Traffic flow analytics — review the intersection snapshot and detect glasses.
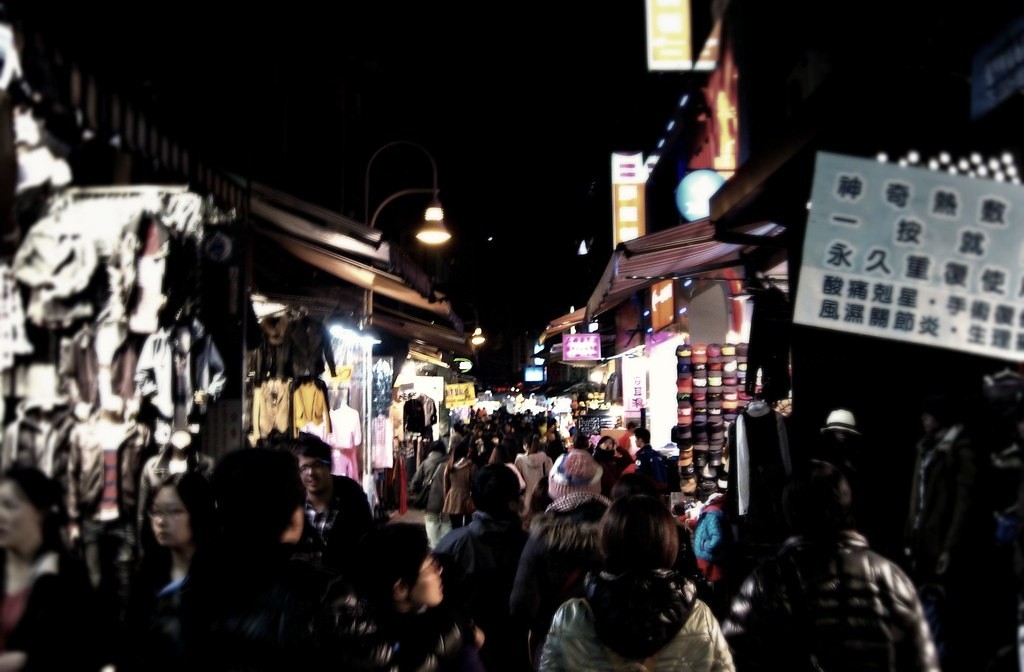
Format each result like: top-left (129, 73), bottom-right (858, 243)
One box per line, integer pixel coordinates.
top-left (298, 460), bottom-right (332, 472)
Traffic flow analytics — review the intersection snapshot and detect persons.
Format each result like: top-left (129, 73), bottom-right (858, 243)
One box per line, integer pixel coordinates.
top-left (138, 430), bottom-right (215, 560)
top-left (0, 404), bottom-right (1023, 671)
top-left (65, 395), bottom-right (152, 609)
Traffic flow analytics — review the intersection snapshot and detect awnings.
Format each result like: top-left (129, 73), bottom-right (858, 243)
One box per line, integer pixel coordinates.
top-left (584, 215), bottom-right (788, 324)
top-left (538, 305), bottom-right (626, 346)
top-left (242, 182), bottom-right (475, 355)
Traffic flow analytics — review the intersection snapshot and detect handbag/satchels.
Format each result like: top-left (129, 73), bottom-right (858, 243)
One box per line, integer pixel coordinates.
top-left (414, 483), bottom-right (431, 509)
top-left (464, 497), bottom-right (475, 512)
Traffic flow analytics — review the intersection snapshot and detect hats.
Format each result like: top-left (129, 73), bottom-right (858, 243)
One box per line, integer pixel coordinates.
top-left (548, 451), bottom-right (605, 499)
top-left (820, 409), bottom-right (864, 439)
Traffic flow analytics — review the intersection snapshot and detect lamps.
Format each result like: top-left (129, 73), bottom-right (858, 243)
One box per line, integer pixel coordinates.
top-left (366, 140), bottom-right (453, 248)
